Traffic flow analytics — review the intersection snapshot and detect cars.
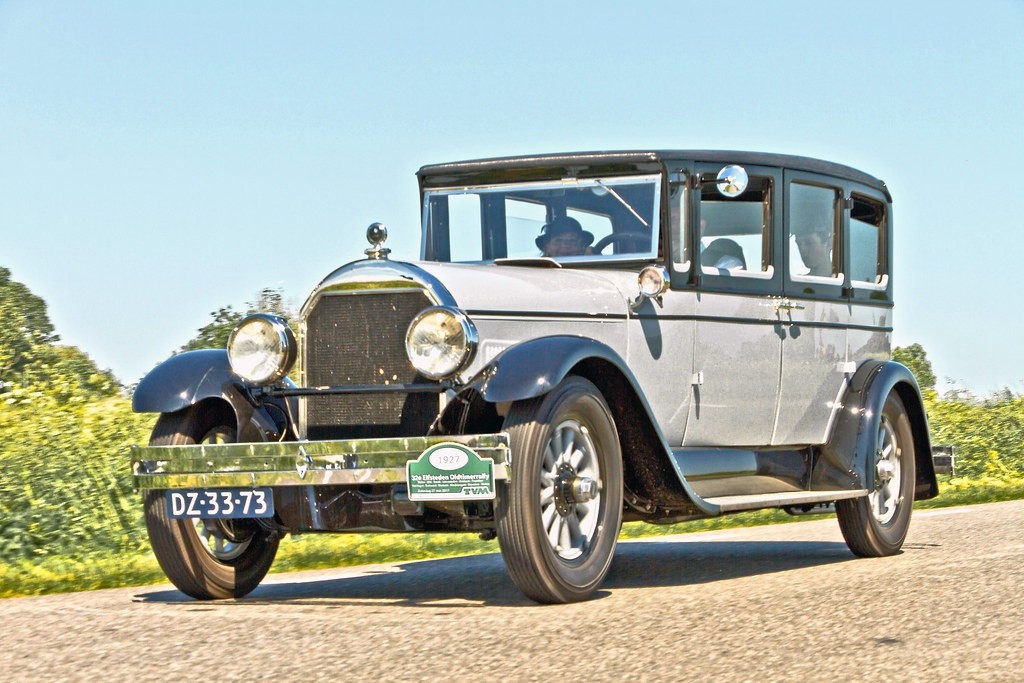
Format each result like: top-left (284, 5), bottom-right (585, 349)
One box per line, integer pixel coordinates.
top-left (128, 148), bottom-right (940, 606)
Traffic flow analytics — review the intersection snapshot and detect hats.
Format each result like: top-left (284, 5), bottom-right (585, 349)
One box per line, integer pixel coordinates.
top-left (535, 217), bottom-right (593, 253)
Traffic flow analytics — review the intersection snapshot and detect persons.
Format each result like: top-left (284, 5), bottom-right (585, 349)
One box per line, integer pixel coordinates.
top-left (790, 206), bottom-right (834, 277)
top-left (670, 195), bottom-right (744, 270)
top-left (535, 216), bottom-right (602, 257)
